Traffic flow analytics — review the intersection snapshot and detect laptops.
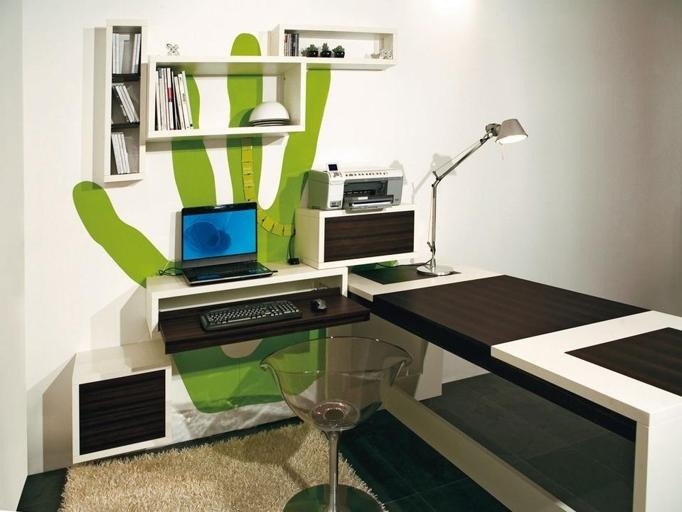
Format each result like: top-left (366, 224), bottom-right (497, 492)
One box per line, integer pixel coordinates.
top-left (181, 202), bottom-right (273, 286)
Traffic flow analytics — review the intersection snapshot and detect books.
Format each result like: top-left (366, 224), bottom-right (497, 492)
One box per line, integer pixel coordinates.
top-left (155, 66), bottom-right (194, 133)
top-left (111, 31), bottom-right (142, 176)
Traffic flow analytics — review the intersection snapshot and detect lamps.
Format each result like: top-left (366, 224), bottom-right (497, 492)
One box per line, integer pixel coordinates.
top-left (416, 118), bottom-right (528, 276)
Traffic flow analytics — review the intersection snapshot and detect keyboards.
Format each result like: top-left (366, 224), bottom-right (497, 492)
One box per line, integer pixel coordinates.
top-left (200, 300), bottom-right (303, 331)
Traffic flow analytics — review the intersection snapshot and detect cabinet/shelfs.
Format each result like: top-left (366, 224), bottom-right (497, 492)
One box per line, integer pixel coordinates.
top-left (146, 54), bottom-right (307, 143)
top-left (70, 330), bottom-right (320, 465)
top-left (270, 22), bottom-right (399, 72)
top-left (145, 263), bottom-right (371, 355)
top-left (294, 206), bottom-right (681, 512)
top-left (100, 16), bottom-right (149, 184)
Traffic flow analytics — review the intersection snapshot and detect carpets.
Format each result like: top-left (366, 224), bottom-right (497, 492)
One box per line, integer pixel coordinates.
top-left (57, 417), bottom-right (389, 512)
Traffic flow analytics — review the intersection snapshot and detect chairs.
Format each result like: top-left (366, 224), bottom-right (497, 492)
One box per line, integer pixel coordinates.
top-left (260, 335), bottom-right (413, 512)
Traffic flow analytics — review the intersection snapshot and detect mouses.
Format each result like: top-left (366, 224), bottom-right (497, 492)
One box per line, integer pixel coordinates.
top-left (313, 298), bottom-right (328, 313)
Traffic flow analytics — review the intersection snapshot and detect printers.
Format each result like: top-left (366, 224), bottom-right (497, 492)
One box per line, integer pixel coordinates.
top-left (307, 164), bottom-right (404, 213)
